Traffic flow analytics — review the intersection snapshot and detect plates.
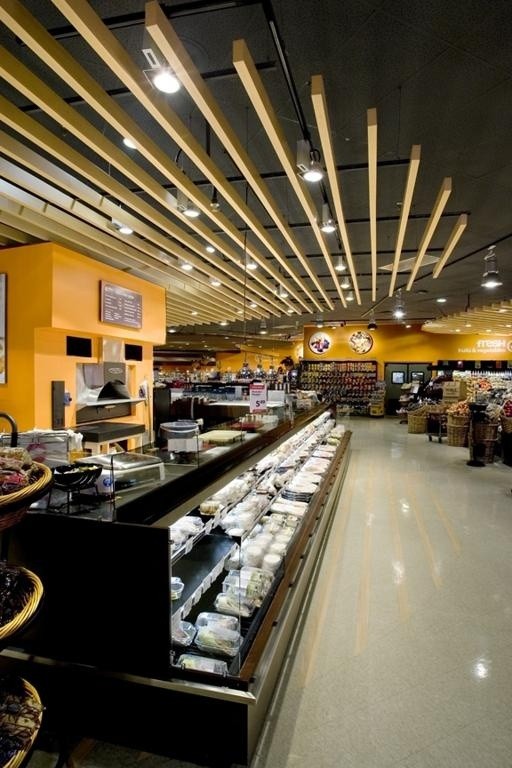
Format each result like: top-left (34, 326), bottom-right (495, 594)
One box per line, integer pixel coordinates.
top-left (270, 444), bottom-right (342, 515)
top-left (228, 422), bottom-right (267, 433)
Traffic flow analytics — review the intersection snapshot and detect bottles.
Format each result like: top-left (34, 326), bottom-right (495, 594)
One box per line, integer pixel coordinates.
top-left (96, 474), bottom-right (115, 493)
top-left (307, 361), bottom-right (373, 372)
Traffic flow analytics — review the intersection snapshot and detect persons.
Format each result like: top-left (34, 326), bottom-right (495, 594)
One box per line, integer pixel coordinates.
top-left (277, 356), bottom-right (299, 390)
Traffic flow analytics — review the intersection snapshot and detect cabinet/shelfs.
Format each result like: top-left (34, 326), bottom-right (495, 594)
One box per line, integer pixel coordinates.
top-left (300, 357), bottom-right (378, 416)
top-left (162, 411), bottom-right (353, 685)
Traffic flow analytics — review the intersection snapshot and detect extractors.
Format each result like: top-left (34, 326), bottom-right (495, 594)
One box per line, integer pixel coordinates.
top-left (79, 364), bottom-right (146, 407)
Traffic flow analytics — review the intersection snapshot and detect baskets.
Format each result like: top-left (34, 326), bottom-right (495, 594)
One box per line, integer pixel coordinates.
top-left (446, 412), bottom-right (512, 463)
top-left (1, 460), bottom-right (48, 529)
top-left (0, 567), bottom-right (43, 652)
top-left (401, 388), bottom-right (411, 395)
top-left (398, 413), bottom-right (408, 420)
top-left (407, 414), bottom-right (428, 433)
top-left (0, 675), bottom-right (43, 768)
top-left (52, 463), bottom-right (103, 488)
top-left (398, 400), bottom-right (409, 407)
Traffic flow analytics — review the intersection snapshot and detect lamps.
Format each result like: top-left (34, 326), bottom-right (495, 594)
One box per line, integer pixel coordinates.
top-left (480, 245), bottom-right (504, 291)
top-left (112, 191), bottom-right (289, 301)
top-left (302, 140), bottom-right (326, 183)
top-left (389, 289), bottom-right (408, 319)
top-left (366, 311), bottom-right (378, 332)
top-left (319, 204), bottom-right (338, 234)
top-left (334, 253), bottom-right (356, 303)
top-left (235, 352), bottom-right (276, 382)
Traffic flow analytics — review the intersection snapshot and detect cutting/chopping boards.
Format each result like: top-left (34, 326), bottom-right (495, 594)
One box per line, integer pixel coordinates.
top-left (196, 429), bottom-right (248, 444)
